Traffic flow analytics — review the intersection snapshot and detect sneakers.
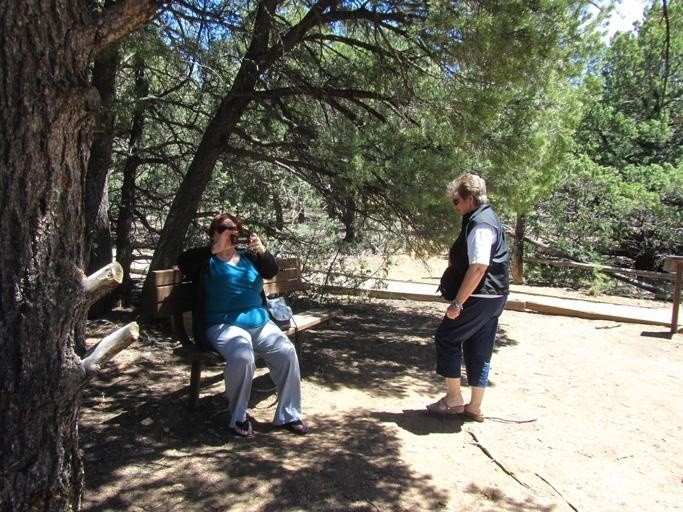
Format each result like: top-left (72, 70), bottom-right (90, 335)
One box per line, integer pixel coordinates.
top-left (282, 418), bottom-right (309, 433)
top-left (427, 398), bottom-right (480, 418)
top-left (235, 416), bottom-right (253, 436)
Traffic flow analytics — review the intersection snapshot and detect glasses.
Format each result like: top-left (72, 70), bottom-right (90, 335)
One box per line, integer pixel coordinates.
top-left (452, 197), bottom-right (462, 205)
top-left (214, 225), bottom-right (237, 234)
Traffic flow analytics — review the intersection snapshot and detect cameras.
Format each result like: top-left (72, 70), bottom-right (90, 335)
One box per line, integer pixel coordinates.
top-left (231, 234), bottom-right (251, 244)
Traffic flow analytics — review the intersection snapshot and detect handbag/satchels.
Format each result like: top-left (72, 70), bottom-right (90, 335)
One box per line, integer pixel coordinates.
top-left (266, 296), bottom-right (293, 331)
top-left (439, 264), bottom-right (487, 301)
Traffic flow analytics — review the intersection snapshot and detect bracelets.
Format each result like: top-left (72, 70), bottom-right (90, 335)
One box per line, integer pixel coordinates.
top-left (453, 299), bottom-right (464, 311)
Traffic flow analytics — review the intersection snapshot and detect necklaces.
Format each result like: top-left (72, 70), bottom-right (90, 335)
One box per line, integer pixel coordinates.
top-left (219, 250), bottom-right (235, 262)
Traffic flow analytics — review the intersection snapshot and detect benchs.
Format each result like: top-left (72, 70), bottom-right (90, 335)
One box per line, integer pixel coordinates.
top-left (142, 257), bottom-right (343, 413)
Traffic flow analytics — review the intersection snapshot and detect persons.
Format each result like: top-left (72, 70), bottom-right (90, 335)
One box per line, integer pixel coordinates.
top-left (425, 173), bottom-right (510, 422)
top-left (175, 212), bottom-right (310, 438)
